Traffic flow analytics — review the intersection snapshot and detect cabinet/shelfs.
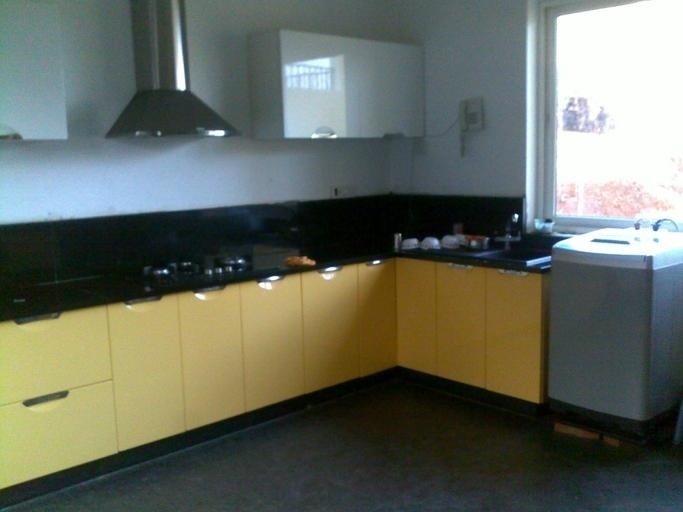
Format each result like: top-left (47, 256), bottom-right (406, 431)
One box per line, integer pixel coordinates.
top-left (250, 27), bottom-right (362, 141)
top-left (359, 256), bottom-right (396, 381)
top-left (245, 261), bottom-right (362, 416)
top-left (1, 306), bottom-right (118, 492)
top-left (391, 255), bottom-right (436, 377)
top-left (362, 36), bottom-right (426, 142)
top-left (0, 0), bottom-right (69, 140)
top-left (437, 259), bottom-right (549, 404)
top-left (108, 279), bottom-right (243, 455)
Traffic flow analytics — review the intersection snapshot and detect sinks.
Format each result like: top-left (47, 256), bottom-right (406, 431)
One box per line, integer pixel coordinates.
top-left (475, 245), bottom-right (554, 264)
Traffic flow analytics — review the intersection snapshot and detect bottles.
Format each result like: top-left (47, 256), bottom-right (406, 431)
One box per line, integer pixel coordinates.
top-left (393, 232), bottom-right (402, 250)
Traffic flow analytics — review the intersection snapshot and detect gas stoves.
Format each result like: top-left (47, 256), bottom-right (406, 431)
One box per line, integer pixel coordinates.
top-left (123, 247), bottom-right (277, 298)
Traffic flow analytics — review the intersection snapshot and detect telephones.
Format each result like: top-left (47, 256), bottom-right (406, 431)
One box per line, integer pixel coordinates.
top-left (458, 96), bottom-right (483, 132)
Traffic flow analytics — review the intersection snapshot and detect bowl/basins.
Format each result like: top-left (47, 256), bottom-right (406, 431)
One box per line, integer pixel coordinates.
top-left (534, 217), bottom-right (556, 234)
top-left (466, 235), bottom-right (489, 250)
top-left (400, 236), bottom-right (419, 251)
top-left (441, 234), bottom-right (459, 250)
top-left (420, 236), bottom-right (442, 250)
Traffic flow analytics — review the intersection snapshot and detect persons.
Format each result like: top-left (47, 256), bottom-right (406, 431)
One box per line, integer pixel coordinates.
top-left (566, 96), bottom-right (609, 134)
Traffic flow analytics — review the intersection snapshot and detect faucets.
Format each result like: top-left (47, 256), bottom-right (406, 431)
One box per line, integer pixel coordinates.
top-left (503, 234), bottom-right (512, 255)
